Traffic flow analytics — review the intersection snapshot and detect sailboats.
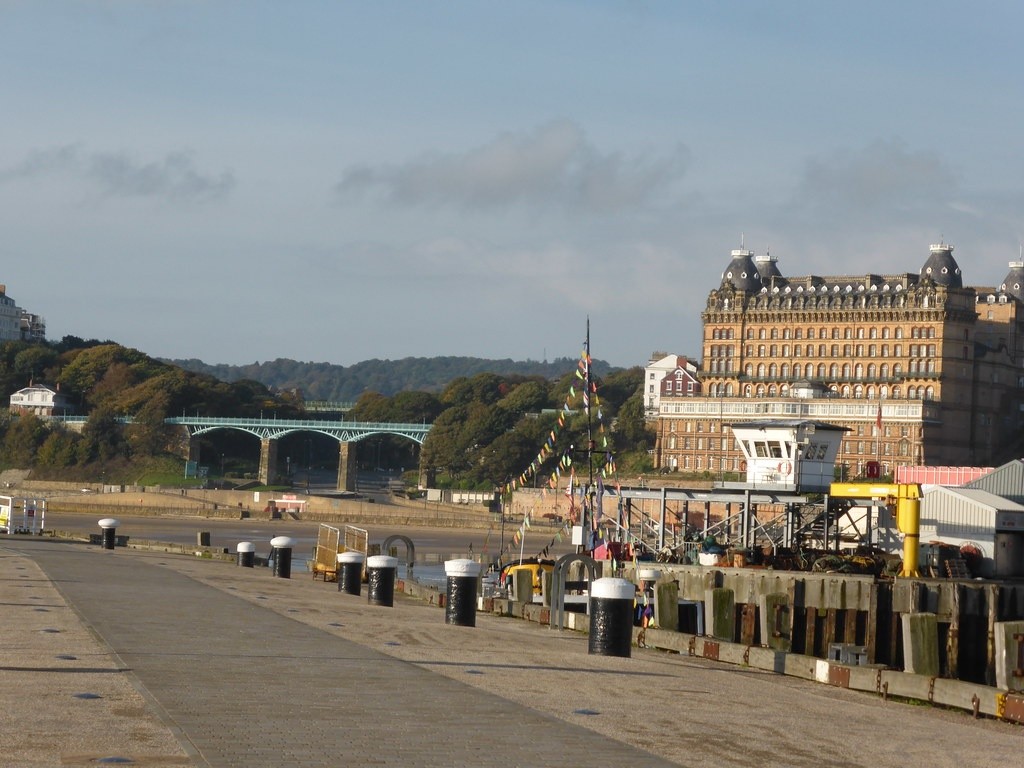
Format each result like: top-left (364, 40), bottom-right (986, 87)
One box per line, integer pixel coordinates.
top-left (498, 314), bottom-right (705, 638)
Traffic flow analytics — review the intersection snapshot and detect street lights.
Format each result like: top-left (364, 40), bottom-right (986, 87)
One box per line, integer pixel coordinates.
top-left (219, 449), bottom-right (225, 479)
top-left (260, 409), bottom-right (263, 419)
top-left (341, 414), bottom-right (344, 421)
top-left (304, 435), bottom-right (313, 494)
top-left (274, 411), bottom-right (276, 419)
top-left (377, 438), bottom-right (383, 472)
top-left (182, 408), bottom-right (186, 422)
top-left (354, 413), bottom-right (357, 422)
top-left (197, 409), bottom-right (199, 418)
top-left (423, 415), bottom-right (426, 424)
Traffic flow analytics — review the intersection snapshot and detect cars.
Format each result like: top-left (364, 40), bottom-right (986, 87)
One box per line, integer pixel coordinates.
top-left (543, 514), bottom-right (563, 523)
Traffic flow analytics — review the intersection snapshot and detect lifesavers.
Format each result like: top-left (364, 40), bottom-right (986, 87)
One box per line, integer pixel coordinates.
top-left (777, 460), bottom-right (791, 477)
top-left (740, 461), bottom-right (747, 471)
